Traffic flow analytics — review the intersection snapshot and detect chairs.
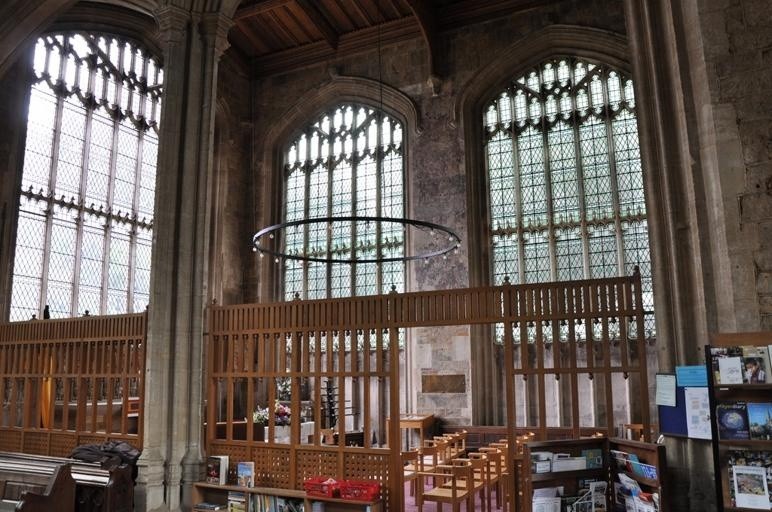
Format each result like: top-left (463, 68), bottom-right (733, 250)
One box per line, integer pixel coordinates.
top-left (402, 429), bottom-right (536, 512)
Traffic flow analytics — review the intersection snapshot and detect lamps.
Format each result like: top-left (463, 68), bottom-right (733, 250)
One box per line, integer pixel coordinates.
top-left (250, 0), bottom-right (461, 267)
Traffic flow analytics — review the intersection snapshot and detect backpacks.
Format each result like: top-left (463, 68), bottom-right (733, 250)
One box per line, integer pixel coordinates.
top-left (67, 438), bottom-right (139, 466)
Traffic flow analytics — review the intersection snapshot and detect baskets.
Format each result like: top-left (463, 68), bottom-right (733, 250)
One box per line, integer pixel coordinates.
top-left (303, 477), bottom-right (344, 498)
top-left (339, 481), bottom-right (380, 501)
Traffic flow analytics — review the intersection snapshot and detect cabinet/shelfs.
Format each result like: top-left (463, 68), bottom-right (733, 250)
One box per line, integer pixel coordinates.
top-left (522, 435), bottom-right (608, 512)
top-left (608, 437), bottom-right (667, 512)
top-left (191, 481), bottom-right (383, 512)
top-left (704, 330), bottom-right (772, 512)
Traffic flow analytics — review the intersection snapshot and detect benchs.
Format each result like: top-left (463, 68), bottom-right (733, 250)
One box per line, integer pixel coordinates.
top-left (0, 450), bottom-right (134, 512)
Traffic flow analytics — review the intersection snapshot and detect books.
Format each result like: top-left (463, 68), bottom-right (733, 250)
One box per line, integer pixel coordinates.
top-left (237, 461), bottom-right (255, 488)
top-left (192, 490), bottom-right (325, 512)
top-left (528, 449), bottom-right (660, 511)
top-left (207, 455), bottom-right (230, 486)
top-left (707, 345), bottom-right (772, 511)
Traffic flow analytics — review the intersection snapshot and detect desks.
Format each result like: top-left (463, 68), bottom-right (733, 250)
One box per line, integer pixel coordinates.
top-left (386, 413), bottom-right (434, 447)
